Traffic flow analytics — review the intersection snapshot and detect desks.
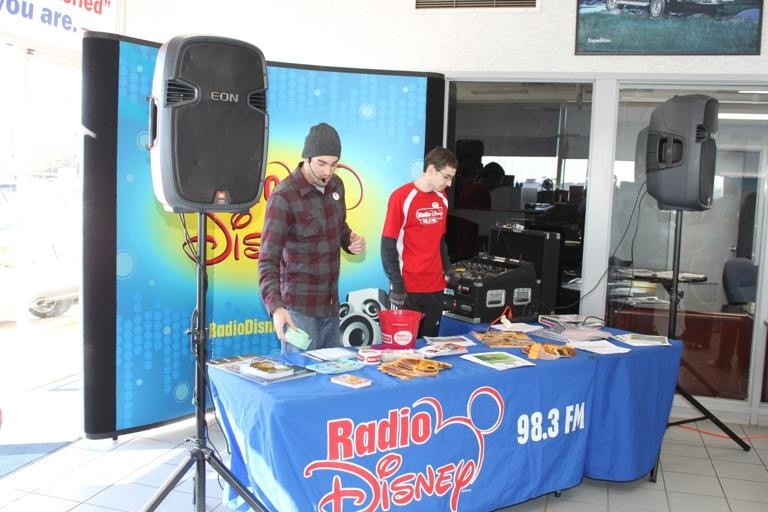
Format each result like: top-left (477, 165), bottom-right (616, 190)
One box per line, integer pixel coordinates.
top-left (207, 316), bottom-right (684, 512)
top-left (611, 304), bottom-right (768, 402)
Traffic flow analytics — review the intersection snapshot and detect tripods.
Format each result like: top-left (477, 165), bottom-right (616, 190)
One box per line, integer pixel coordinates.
top-left (635, 214), bottom-right (753, 483)
top-left (141, 218), bottom-right (271, 512)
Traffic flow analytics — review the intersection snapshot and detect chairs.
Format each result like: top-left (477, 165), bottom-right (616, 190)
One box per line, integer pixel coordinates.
top-left (723, 254), bottom-right (759, 306)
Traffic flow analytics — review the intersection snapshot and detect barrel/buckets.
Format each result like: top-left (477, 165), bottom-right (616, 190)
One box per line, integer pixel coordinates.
top-left (378, 310), bottom-right (426, 349)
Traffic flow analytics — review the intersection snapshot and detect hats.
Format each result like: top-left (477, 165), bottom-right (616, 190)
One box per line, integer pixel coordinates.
top-left (302, 123), bottom-right (341, 158)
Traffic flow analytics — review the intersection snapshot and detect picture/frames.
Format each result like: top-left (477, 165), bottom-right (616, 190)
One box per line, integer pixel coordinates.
top-left (574, 0), bottom-right (762, 54)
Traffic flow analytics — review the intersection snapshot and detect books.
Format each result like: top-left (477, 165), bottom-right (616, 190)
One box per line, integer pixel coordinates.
top-left (239, 360), bottom-right (295, 380)
top-left (330, 373), bottom-right (371, 390)
top-left (628, 333), bottom-right (665, 344)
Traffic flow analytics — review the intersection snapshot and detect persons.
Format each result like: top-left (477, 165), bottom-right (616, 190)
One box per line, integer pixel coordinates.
top-left (450, 138), bottom-right (503, 262)
top-left (381, 146), bottom-right (460, 341)
top-left (257, 123), bottom-right (366, 356)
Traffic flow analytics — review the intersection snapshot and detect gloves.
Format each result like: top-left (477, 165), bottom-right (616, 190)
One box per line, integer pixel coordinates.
top-left (443, 267), bottom-right (459, 287)
top-left (388, 280), bottom-right (407, 309)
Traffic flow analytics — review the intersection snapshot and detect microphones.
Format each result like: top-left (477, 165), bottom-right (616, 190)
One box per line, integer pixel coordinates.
top-left (306, 158), bottom-right (327, 188)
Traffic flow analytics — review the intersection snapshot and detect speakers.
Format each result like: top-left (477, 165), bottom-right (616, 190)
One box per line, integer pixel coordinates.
top-left (337, 288), bottom-right (391, 348)
top-left (643, 95), bottom-right (721, 211)
top-left (147, 32), bottom-right (271, 214)
